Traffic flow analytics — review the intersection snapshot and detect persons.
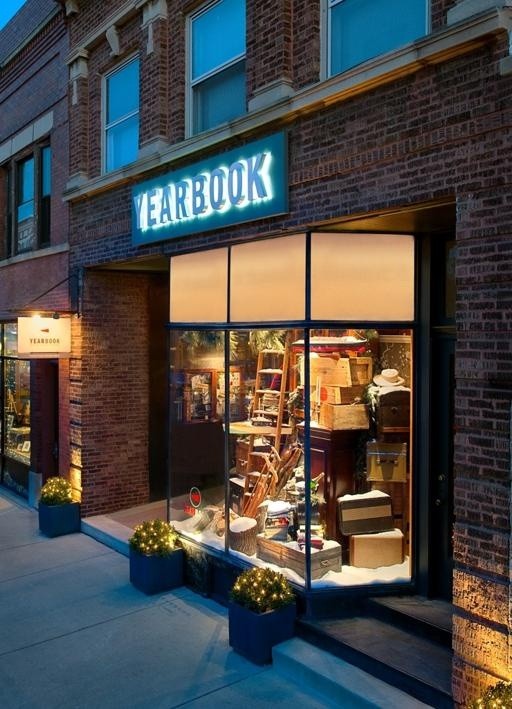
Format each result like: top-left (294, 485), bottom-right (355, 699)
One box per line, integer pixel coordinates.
top-left (18, 398), bottom-right (30, 429)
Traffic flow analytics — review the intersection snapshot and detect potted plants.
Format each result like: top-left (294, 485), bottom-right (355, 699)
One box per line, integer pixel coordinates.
top-left (226, 565), bottom-right (298, 666)
top-left (127, 515), bottom-right (186, 597)
top-left (36, 474), bottom-right (82, 538)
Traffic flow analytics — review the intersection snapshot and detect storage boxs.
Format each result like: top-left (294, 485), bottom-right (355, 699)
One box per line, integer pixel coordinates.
top-left (224, 341), bottom-right (411, 580)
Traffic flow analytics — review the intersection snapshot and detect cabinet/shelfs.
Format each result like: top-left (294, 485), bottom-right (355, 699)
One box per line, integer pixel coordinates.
top-left (1, 356), bottom-right (61, 500)
top-left (178, 364), bottom-right (246, 427)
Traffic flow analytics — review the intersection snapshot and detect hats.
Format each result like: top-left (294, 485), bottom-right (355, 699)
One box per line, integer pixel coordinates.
top-left (373, 369), bottom-right (405, 386)
